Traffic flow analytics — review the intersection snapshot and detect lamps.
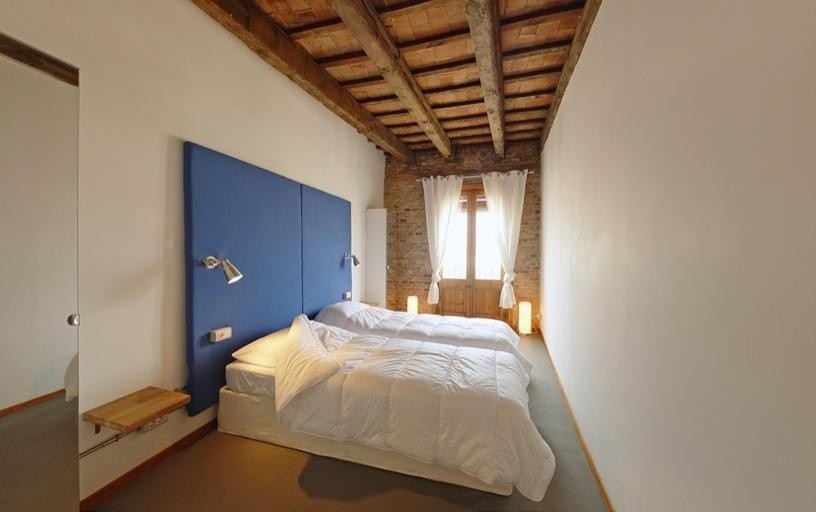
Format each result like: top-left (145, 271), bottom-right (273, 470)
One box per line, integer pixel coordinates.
top-left (407, 296), bottom-right (420, 314)
top-left (518, 302), bottom-right (532, 334)
top-left (344, 254), bottom-right (362, 267)
top-left (204, 255), bottom-right (243, 286)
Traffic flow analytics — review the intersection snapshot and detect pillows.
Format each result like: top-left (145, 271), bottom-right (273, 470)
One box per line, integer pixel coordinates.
top-left (231, 325), bottom-right (291, 368)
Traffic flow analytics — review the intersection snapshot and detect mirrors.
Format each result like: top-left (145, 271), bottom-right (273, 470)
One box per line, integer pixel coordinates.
top-left (0, 32), bottom-right (82, 512)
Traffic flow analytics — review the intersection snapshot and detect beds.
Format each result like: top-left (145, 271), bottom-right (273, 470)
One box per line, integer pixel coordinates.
top-left (313, 301), bottom-right (534, 381)
top-left (214, 315), bottom-right (556, 502)
